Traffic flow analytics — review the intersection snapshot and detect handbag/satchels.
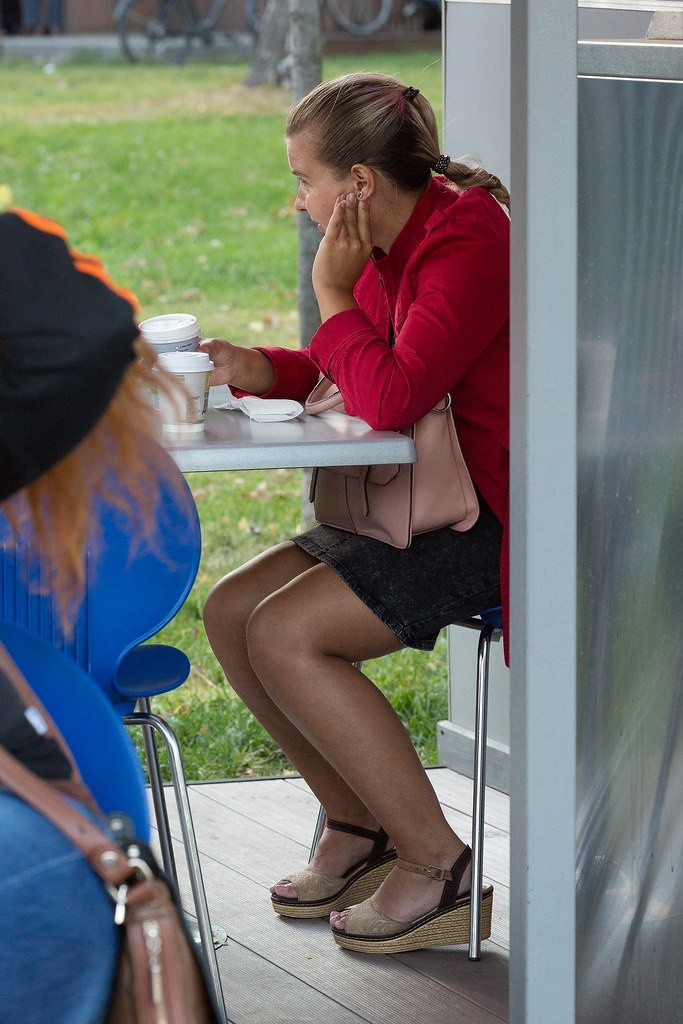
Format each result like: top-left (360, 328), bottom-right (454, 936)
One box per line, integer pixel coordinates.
top-left (90, 839), bottom-right (212, 1024)
top-left (304, 375), bottom-right (479, 550)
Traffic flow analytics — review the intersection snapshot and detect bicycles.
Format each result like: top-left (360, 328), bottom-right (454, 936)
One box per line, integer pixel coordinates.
top-left (117, 1), bottom-right (267, 67)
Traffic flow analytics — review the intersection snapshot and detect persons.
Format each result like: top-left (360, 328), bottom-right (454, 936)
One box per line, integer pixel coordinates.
top-left (0, 208), bottom-right (190, 1024)
top-left (196, 75), bottom-right (510, 954)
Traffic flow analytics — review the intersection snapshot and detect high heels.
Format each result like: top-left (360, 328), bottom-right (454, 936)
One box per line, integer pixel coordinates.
top-left (270, 816), bottom-right (398, 918)
top-left (328, 843), bottom-right (494, 954)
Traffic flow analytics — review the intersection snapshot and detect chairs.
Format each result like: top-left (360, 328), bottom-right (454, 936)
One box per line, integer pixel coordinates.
top-left (0, 425), bottom-right (228, 1024)
top-left (303, 604), bottom-right (507, 958)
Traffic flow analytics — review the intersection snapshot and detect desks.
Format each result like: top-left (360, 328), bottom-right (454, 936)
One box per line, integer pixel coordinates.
top-left (134, 417), bottom-right (420, 962)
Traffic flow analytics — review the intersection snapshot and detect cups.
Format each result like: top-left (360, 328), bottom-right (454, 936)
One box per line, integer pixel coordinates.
top-left (138, 312), bottom-right (202, 412)
top-left (151, 351), bottom-right (215, 433)
